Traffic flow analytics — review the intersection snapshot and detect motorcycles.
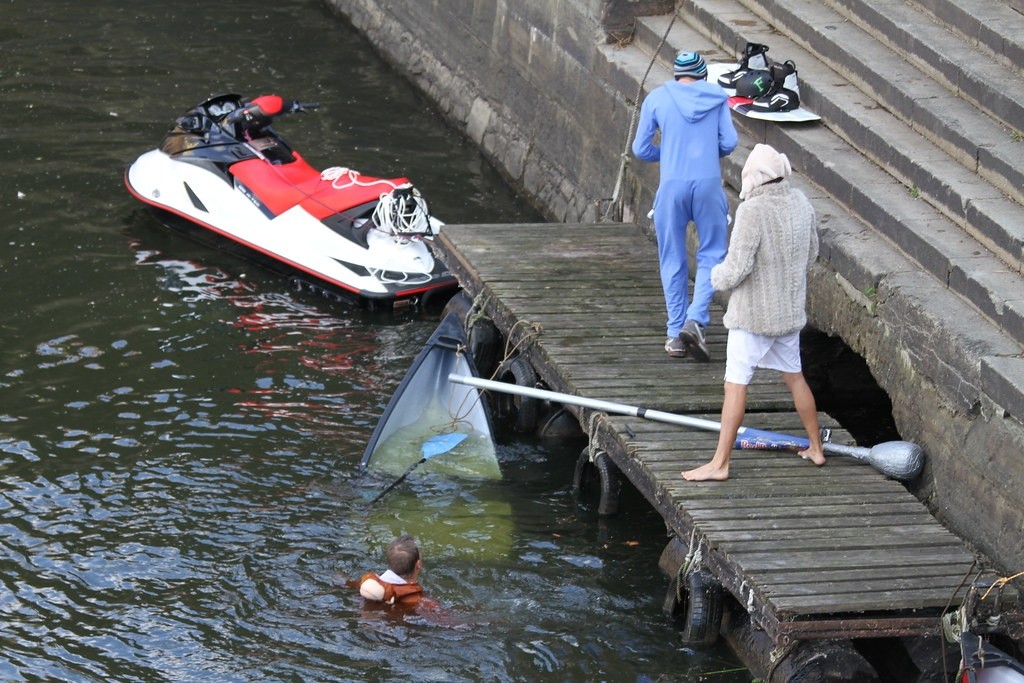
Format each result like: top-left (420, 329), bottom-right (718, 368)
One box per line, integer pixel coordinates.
top-left (123, 91), bottom-right (462, 314)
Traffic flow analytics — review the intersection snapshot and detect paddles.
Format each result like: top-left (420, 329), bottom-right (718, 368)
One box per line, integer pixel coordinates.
top-left (366, 432), bottom-right (470, 505)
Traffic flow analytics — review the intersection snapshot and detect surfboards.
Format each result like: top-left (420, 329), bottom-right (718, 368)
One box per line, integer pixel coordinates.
top-left (704, 64), bottom-right (823, 123)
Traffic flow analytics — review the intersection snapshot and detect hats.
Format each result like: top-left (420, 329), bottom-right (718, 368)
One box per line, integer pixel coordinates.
top-left (739, 143), bottom-right (792, 199)
top-left (674, 51), bottom-right (708, 81)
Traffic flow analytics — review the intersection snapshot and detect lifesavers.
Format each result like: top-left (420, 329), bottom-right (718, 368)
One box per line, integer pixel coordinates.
top-left (572, 446), bottom-right (624, 523)
top-left (460, 311), bottom-right (501, 376)
top-left (661, 563), bottom-right (725, 649)
top-left (490, 359), bottom-right (543, 429)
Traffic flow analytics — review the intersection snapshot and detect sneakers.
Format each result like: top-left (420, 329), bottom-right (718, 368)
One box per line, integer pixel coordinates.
top-left (679, 320), bottom-right (711, 361)
top-left (718, 42), bottom-right (769, 88)
top-left (664, 337), bottom-right (688, 357)
top-left (752, 60), bottom-right (800, 112)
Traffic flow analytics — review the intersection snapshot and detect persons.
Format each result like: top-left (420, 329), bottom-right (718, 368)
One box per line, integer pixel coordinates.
top-left (632, 51), bottom-right (738, 361)
top-left (682, 143), bottom-right (826, 480)
top-left (359, 535), bottom-right (424, 603)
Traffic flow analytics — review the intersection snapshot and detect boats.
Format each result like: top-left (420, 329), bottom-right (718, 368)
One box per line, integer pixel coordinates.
top-left (348, 306), bottom-right (518, 566)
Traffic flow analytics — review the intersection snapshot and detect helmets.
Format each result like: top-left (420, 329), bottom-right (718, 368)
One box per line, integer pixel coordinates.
top-left (736, 70), bottom-right (776, 99)
